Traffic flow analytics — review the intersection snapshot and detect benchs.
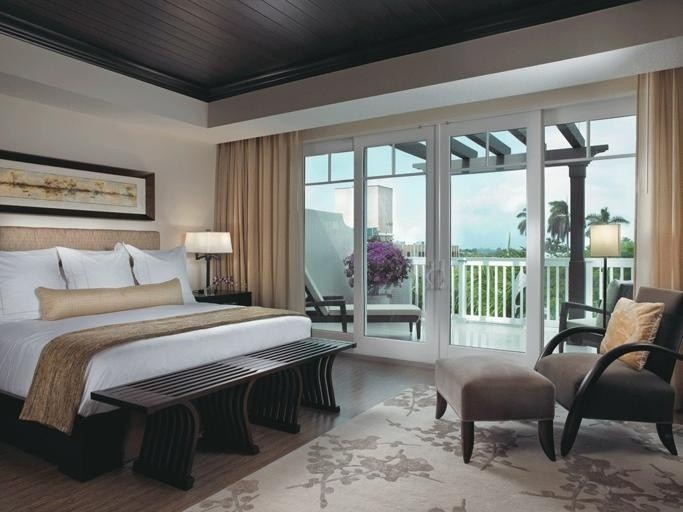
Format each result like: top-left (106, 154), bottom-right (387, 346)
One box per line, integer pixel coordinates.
top-left (91, 337), bottom-right (356, 490)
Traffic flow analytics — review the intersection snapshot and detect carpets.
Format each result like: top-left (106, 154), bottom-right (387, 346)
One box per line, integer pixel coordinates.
top-left (182, 383), bottom-right (683, 512)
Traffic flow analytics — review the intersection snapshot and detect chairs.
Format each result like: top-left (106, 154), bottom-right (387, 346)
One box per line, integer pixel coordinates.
top-left (535, 287), bottom-right (683, 456)
top-left (303, 269), bottom-right (422, 340)
top-left (559, 282), bottom-right (633, 353)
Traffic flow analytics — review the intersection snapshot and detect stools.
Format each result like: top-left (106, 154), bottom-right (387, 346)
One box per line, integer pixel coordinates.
top-left (434, 354), bottom-right (554, 463)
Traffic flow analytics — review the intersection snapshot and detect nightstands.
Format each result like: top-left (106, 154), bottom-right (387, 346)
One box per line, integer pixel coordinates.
top-left (193, 290), bottom-right (251, 306)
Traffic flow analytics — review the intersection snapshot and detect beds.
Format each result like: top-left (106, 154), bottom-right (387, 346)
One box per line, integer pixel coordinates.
top-left (0, 227), bottom-right (312, 482)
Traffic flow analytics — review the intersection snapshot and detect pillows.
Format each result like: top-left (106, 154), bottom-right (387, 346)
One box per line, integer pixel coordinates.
top-left (36, 278), bottom-right (183, 322)
top-left (123, 241), bottom-right (197, 305)
top-left (600, 297), bottom-right (665, 371)
top-left (596, 280), bottom-right (632, 329)
top-left (0, 248), bottom-right (65, 321)
top-left (58, 242), bottom-right (136, 290)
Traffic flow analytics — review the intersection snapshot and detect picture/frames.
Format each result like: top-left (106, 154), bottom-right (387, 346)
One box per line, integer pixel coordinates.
top-left (0, 149), bottom-right (154, 220)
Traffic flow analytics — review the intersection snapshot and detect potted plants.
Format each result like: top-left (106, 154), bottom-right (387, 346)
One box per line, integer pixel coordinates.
top-left (344, 241), bottom-right (409, 304)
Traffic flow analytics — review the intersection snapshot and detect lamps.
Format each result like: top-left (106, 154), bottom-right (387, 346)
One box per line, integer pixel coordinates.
top-left (184, 228), bottom-right (233, 294)
top-left (590, 223), bottom-right (618, 330)
